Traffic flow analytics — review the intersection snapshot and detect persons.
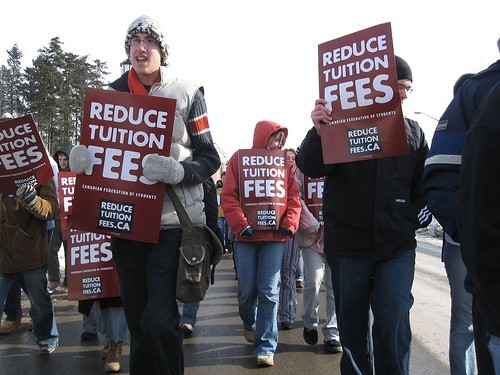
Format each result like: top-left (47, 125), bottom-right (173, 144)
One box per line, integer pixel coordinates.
top-left (46, 145), bottom-right (73, 293)
top-left (221, 121), bottom-right (301, 366)
top-left (292, 164), bottom-right (342, 353)
top-left (0, 113), bottom-right (59, 354)
top-left (178, 176), bottom-right (219, 335)
top-left (70, 14), bottom-right (221, 375)
top-left (426, 38), bottom-right (500, 375)
top-left (216, 171), bottom-right (232, 257)
top-left (295, 54), bottom-right (430, 375)
top-left (78, 296), bottom-right (132, 373)
top-left (276, 147), bottom-right (306, 330)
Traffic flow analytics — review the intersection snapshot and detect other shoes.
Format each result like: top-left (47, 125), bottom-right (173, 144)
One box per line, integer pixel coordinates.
top-left (283, 325), bottom-right (293, 330)
top-left (64, 278), bottom-right (67, 287)
top-left (303, 327), bottom-right (318, 345)
top-left (0, 320), bottom-right (21, 334)
top-left (81, 332), bottom-right (97, 341)
top-left (295, 281), bottom-right (303, 289)
top-left (102, 343), bottom-right (112, 359)
top-left (324, 340), bottom-right (343, 352)
top-left (39, 346), bottom-right (55, 354)
top-left (104, 342), bottom-right (122, 372)
top-left (182, 323), bottom-right (193, 336)
top-left (47, 281), bottom-right (60, 294)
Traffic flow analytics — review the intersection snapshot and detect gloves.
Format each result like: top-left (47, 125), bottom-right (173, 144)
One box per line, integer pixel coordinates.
top-left (69, 145), bottom-right (90, 174)
top-left (239, 226), bottom-right (254, 237)
top-left (143, 154), bottom-right (185, 185)
top-left (278, 228), bottom-right (292, 236)
top-left (16, 185), bottom-right (37, 204)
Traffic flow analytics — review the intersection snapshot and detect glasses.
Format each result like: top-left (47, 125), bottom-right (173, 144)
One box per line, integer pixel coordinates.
top-left (398, 85), bottom-right (413, 94)
top-left (128, 36), bottom-right (158, 47)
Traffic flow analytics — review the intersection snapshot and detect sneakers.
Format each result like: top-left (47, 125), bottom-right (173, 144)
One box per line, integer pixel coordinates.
top-left (244, 329), bottom-right (254, 342)
top-left (256, 354), bottom-right (274, 366)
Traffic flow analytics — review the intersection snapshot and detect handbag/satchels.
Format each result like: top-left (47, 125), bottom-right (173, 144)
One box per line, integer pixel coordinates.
top-left (176, 223), bottom-right (224, 305)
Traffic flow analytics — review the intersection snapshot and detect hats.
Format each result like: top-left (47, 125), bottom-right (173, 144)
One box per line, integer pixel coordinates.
top-left (453, 74), bottom-right (476, 97)
top-left (216, 180), bottom-right (223, 188)
top-left (120, 15), bottom-right (170, 67)
top-left (395, 55), bottom-right (413, 83)
